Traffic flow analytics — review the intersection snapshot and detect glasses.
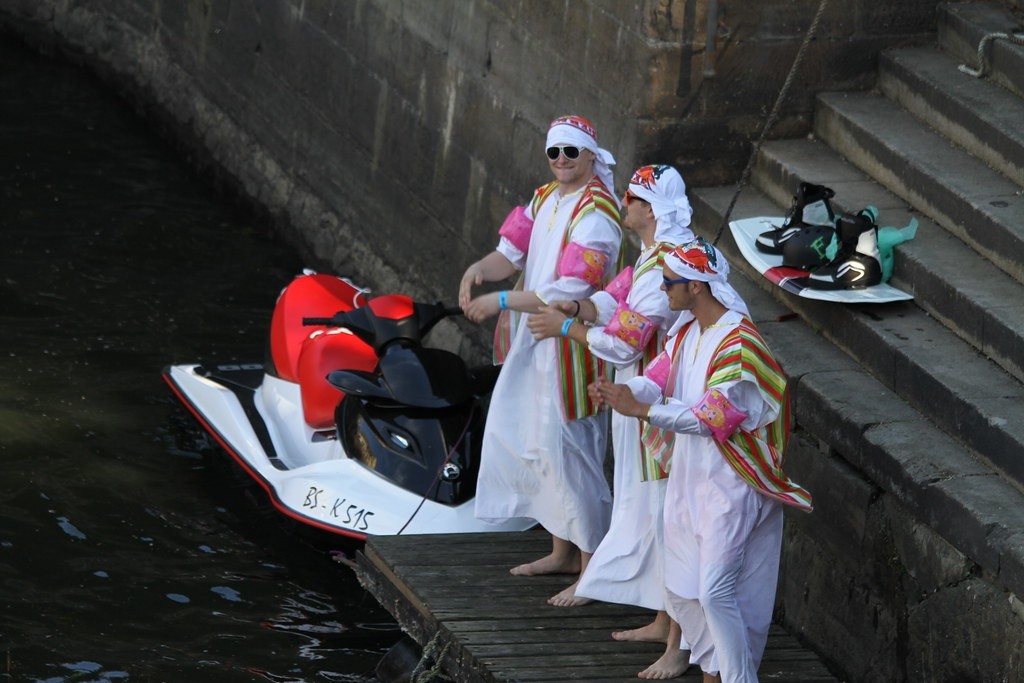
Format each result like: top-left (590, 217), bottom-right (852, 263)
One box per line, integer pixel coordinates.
top-left (625, 189), bottom-right (647, 204)
top-left (663, 274), bottom-right (693, 287)
top-left (545, 145), bottom-right (585, 161)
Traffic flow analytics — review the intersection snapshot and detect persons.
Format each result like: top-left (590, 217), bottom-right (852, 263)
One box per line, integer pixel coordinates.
top-left (588, 240), bottom-right (815, 683)
top-left (459, 115), bottom-right (624, 607)
top-left (527, 165), bottom-right (695, 680)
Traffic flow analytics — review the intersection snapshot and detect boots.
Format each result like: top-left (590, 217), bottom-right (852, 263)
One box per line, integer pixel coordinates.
top-left (806, 209), bottom-right (883, 291)
top-left (755, 179), bottom-right (836, 255)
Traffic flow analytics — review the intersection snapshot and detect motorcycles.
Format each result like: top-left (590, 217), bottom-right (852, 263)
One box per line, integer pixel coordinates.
top-left (160, 264), bottom-right (543, 552)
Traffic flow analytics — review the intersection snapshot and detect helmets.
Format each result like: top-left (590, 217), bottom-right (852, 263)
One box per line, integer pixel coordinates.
top-left (782, 226), bottom-right (841, 272)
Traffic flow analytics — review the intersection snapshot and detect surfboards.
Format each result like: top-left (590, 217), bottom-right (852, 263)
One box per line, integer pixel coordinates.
top-left (729, 215), bottom-right (915, 303)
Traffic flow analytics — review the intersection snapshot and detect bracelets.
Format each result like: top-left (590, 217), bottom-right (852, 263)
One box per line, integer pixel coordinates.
top-left (499, 290), bottom-right (508, 310)
top-left (573, 300), bottom-right (580, 317)
top-left (561, 318), bottom-right (573, 336)
